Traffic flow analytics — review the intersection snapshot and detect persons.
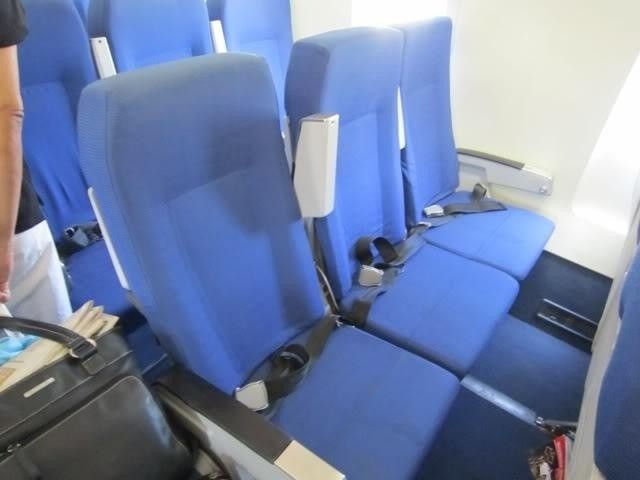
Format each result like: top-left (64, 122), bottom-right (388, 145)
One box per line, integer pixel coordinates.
top-left (1, 0), bottom-right (74, 340)
